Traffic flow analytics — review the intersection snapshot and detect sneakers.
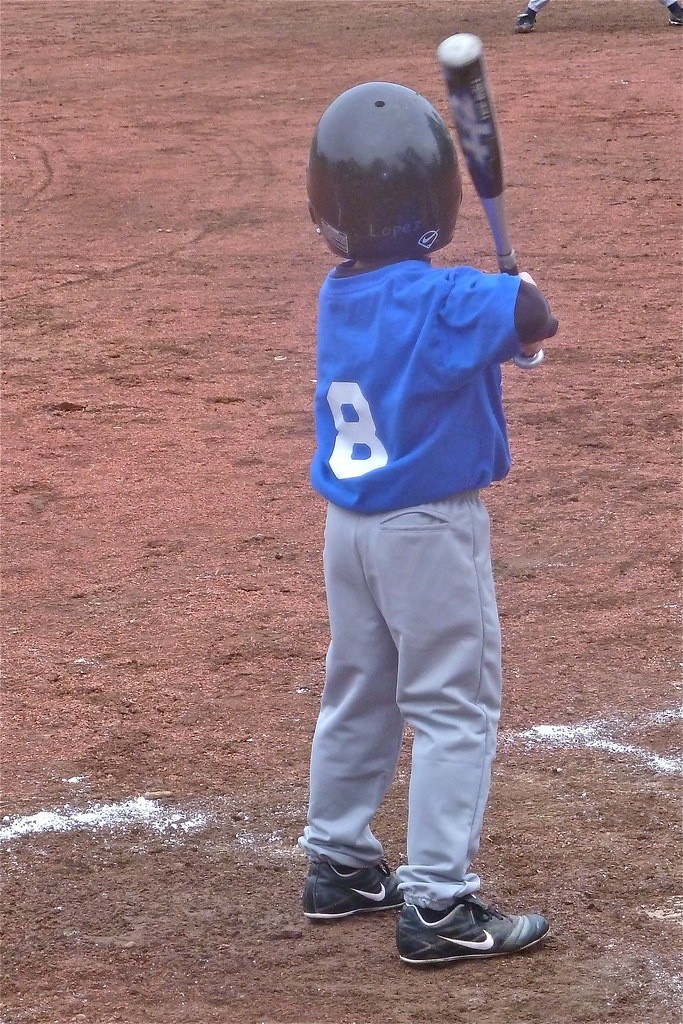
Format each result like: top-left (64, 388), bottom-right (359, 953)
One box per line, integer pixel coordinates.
top-left (301, 857), bottom-right (405, 918)
top-left (668, 14), bottom-right (683, 26)
top-left (513, 13), bottom-right (537, 33)
top-left (395, 900), bottom-right (552, 967)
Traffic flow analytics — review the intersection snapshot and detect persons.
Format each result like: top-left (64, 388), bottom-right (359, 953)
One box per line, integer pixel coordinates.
top-left (513, 0), bottom-right (682, 48)
top-left (295, 75), bottom-right (562, 966)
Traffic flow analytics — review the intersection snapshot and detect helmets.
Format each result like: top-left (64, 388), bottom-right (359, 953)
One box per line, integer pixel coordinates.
top-left (307, 82), bottom-right (464, 257)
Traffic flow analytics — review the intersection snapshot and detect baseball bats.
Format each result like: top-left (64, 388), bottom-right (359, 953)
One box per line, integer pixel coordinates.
top-left (436, 32), bottom-right (547, 371)
